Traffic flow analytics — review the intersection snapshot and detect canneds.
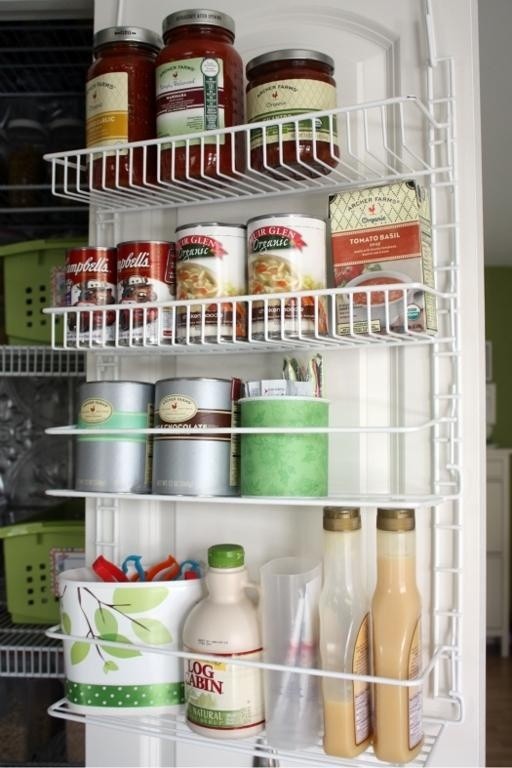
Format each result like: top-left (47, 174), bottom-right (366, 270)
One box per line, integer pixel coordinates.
top-left (247, 213), bottom-right (328, 340)
top-left (84, 27), bottom-right (164, 201)
top-left (64, 246), bottom-right (116, 347)
top-left (116, 240), bottom-right (175, 346)
top-left (153, 9), bottom-right (244, 190)
top-left (245, 47), bottom-right (340, 182)
top-left (174, 221), bottom-right (247, 344)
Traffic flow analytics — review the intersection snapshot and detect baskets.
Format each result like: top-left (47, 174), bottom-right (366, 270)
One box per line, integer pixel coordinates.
top-left (1, 239), bottom-right (88, 348)
top-left (0, 519), bottom-right (85, 624)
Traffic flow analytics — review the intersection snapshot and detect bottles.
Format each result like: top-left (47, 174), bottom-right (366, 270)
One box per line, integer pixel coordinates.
top-left (319, 507), bottom-right (372, 755)
top-left (182, 543), bottom-right (265, 733)
top-left (375, 511), bottom-right (426, 760)
